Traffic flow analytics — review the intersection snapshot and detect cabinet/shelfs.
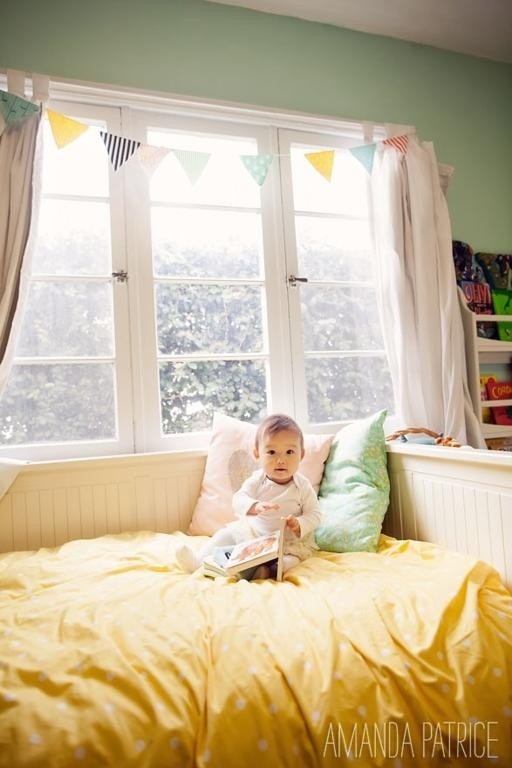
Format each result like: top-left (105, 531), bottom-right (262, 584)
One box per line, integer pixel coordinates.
top-left (457, 283), bottom-right (511, 441)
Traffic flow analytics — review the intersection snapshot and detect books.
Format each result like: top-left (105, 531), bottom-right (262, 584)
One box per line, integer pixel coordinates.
top-left (447, 236), bottom-right (512, 455)
top-left (202, 516), bottom-right (292, 583)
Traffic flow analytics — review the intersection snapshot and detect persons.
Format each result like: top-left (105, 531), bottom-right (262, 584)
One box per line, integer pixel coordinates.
top-left (200, 411), bottom-right (321, 580)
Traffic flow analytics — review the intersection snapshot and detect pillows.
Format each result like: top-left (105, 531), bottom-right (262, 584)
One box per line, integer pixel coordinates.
top-left (187, 408), bottom-right (390, 554)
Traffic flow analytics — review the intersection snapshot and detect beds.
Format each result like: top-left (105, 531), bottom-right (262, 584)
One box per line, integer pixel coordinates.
top-left (2, 442), bottom-right (512, 768)
